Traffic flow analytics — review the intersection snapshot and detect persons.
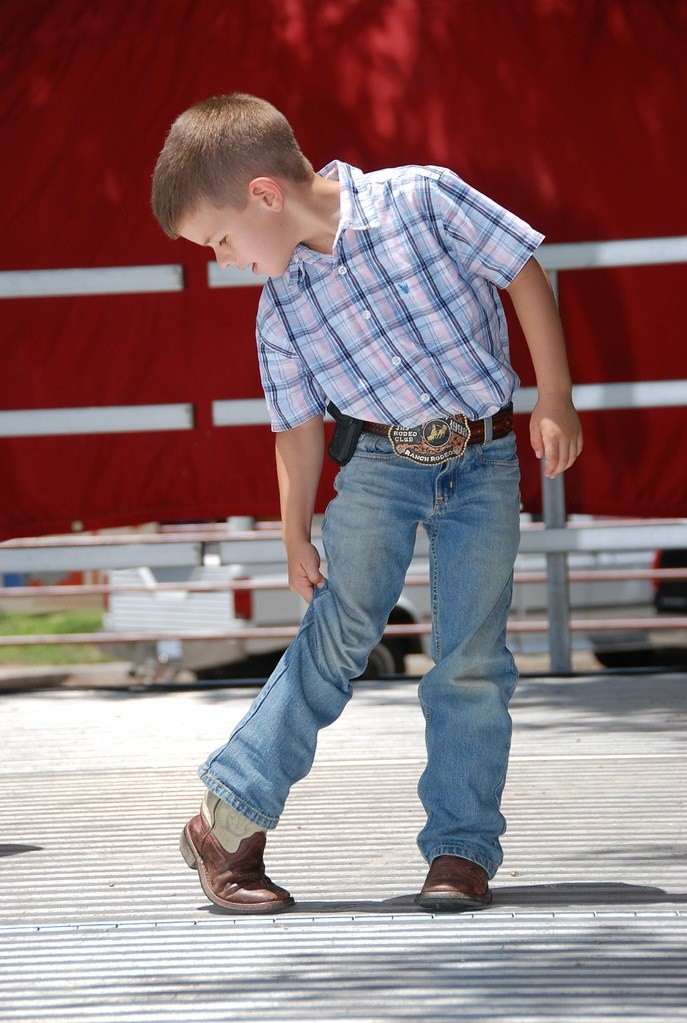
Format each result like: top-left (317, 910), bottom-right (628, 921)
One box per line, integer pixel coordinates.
top-left (151, 92), bottom-right (583, 915)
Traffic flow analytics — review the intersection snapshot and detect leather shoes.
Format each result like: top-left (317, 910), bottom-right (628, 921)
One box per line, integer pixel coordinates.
top-left (178, 788), bottom-right (294, 912)
top-left (413, 856), bottom-right (491, 910)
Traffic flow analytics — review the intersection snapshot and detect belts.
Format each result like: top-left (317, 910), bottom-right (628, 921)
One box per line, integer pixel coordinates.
top-left (359, 400), bottom-right (514, 453)
